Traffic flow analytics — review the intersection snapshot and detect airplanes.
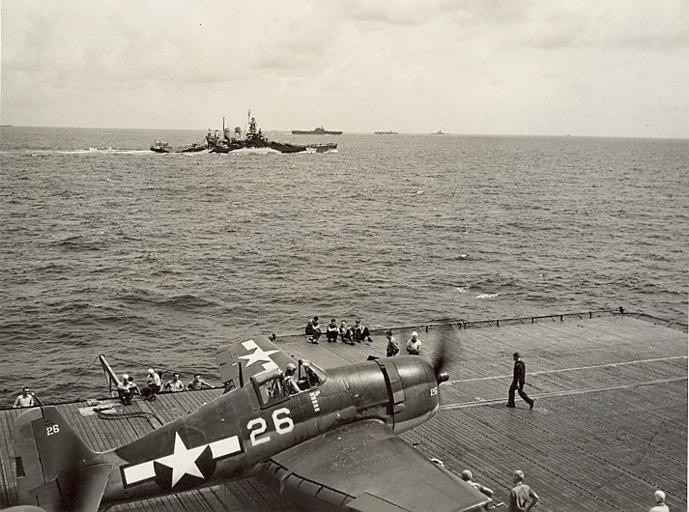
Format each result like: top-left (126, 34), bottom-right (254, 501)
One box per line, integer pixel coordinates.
top-left (12, 308), bottom-right (494, 512)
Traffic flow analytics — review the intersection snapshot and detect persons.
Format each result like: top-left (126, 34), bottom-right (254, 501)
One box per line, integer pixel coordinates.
top-left (117, 369), bottom-right (185, 406)
top-left (281, 363), bottom-right (310, 397)
top-left (326, 319), bottom-right (340, 342)
top-left (185, 373), bottom-right (215, 391)
top-left (406, 331), bottom-right (422, 355)
top-left (649, 490), bottom-right (669, 512)
top-left (384, 330), bottom-right (400, 357)
top-left (461, 470), bottom-right (496, 511)
top-left (506, 470), bottom-right (539, 512)
top-left (351, 318), bottom-right (373, 343)
top-left (305, 316), bottom-right (322, 344)
top-left (13, 387), bottom-right (35, 408)
top-left (337, 320), bottom-right (355, 345)
top-left (506, 352), bottom-right (534, 409)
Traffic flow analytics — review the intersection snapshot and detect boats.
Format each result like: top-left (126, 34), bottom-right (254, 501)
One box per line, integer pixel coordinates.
top-left (150, 108), bottom-right (337, 154)
top-left (429, 130), bottom-right (450, 135)
top-left (374, 130), bottom-right (398, 135)
top-left (292, 125), bottom-right (342, 135)
